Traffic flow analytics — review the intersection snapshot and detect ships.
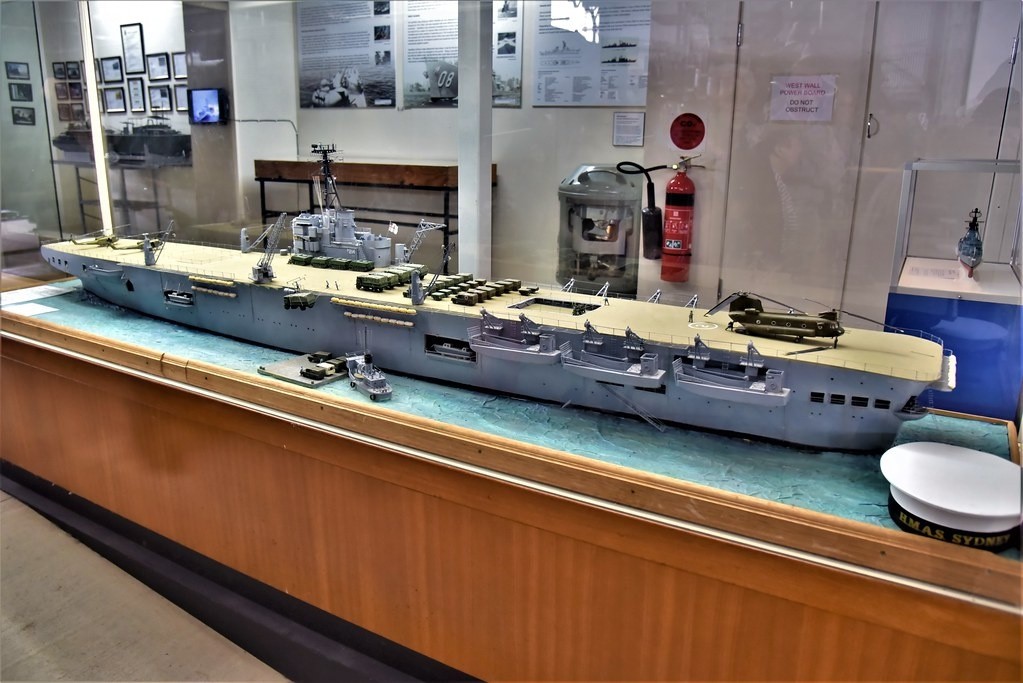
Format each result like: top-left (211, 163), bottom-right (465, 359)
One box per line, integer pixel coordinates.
top-left (38, 144), bottom-right (959, 454)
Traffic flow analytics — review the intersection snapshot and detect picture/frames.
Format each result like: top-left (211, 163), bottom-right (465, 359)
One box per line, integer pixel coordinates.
top-left (5, 62), bottom-right (30, 80)
top-left (147, 85), bottom-right (173, 112)
top-left (12, 106), bottom-right (35, 126)
top-left (103, 87), bottom-right (126, 113)
top-left (80, 58), bottom-right (102, 84)
top-left (68, 82), bottom-right (83, 100)
top-left (127, 77), bottom-right (145, 113)
top-left (145, 52), bottom-right (171, 81)
top-left (8, 83), bottom-right (33, 102)
top-left (55, 82), bottom-right (69, 100)
top-left (100, 56), bottom-right (124, 83)
top-left (172, 52), bottom-right (188, 80)
top-left (70, 103), bottom-right (85, 121)
top-left (66, 61), bottom-right (80, 80)
top-left (174, 84), bottom-right (188, 111)
top-left (120, 23), bottom-right (146, 75)
top-left (84, 89), bottom-right (105, 113)
top-left (52, 62), bottom-right (67, 79)
top-left (57, 103), bottom-right (71, 121)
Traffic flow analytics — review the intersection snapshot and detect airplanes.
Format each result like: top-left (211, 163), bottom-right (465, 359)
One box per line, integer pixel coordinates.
top-left (727, 295), bottom-right (846, 344)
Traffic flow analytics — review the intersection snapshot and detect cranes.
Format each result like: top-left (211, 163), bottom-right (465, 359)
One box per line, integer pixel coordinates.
top-left (245, 211), bottom-right (287, 284)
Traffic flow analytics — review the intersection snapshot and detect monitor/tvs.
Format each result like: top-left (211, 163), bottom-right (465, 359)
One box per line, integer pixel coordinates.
top-left (187, 87), bottom-right (228, 125)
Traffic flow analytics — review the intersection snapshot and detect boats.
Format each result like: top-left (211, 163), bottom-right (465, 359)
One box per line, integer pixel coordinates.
top-left (345, 354), bottom-right (392, 399)
top-left (52, 114), bottom-right (191, 157)
top-left (672, 357), bottom-right (792, 408)
top-left (468, 331), bottom-right (562, 366)
top-left (560, 349), bottom-right (667, 390)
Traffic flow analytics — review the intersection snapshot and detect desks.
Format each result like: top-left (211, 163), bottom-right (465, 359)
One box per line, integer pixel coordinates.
top-left (252, 159), bottom-right (499, 277)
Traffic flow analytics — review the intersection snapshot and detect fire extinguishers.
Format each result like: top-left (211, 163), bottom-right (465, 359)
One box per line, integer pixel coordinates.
top-left (616, 154), bottom-right (706, 283)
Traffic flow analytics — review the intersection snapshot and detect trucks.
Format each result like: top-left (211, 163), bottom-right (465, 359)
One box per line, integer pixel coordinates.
top-left (355, 262), bottom-right (540, 306)
top-left (299, 350), bottom-right (347, 381)
